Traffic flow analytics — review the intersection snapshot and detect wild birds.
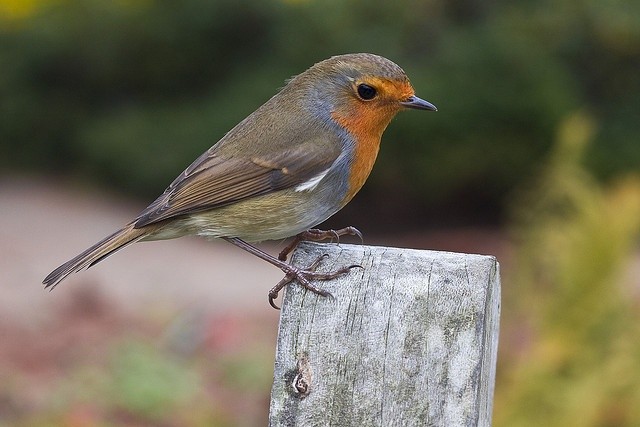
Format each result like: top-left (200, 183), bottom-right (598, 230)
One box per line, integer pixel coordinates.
top-left (42, 48), bottom-right (438, 312)
